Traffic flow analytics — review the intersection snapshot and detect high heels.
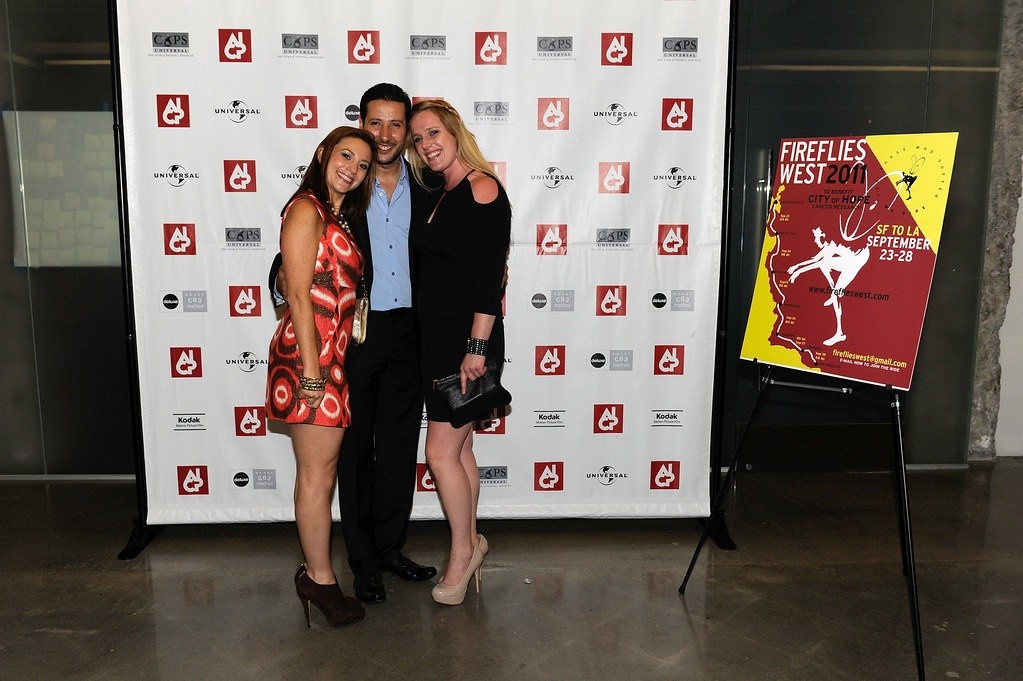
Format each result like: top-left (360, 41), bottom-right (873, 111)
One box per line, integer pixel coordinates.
top-left (293, 564), bottom-right (366, 628)
top-left (438, 533), bottom-right (488, 584)
top-left (432, 542), bottom-right (484, 605)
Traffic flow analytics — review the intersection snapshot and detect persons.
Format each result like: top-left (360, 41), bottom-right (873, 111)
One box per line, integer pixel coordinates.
top-left (264, 126), bottom-right (377, 627)
top-left (408, 101), bottom-right (511, 605)
top-left (269, 83), bottom-right (508, 604)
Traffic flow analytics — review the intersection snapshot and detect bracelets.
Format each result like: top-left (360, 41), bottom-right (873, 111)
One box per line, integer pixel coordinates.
top-left (299, 377), bottom-right (325, 390)
top-left (467, 338), bottom-right (488, 355)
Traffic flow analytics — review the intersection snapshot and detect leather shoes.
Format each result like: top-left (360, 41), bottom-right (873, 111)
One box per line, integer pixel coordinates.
top-left (383, 550), bottom-right (437, 582)
top-left (348, 560), bottom-right (385, 605)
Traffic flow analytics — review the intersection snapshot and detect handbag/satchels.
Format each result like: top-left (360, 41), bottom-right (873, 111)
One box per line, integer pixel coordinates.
top-left (351, 297), bottom-right (368, 343)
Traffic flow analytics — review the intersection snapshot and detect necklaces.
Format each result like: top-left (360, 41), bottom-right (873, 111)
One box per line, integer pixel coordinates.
top-left (326, 201), bottom-right (352, 234)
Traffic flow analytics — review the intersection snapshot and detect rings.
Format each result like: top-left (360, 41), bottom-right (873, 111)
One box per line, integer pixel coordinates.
top-left (307, 399), bottom-right (313, 405)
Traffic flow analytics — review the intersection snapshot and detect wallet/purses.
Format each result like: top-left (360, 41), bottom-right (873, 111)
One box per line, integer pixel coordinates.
top-left (433, 366), bottom-right (513, 429)
